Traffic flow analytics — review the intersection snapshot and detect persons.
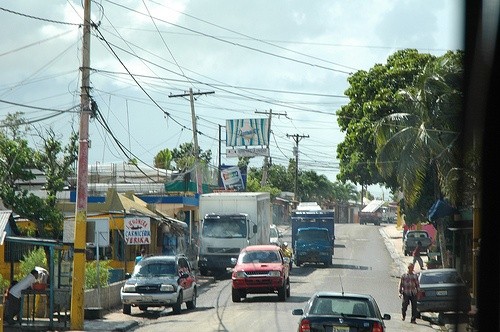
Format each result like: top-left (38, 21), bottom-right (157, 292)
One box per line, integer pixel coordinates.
top-left (247, 252), bottom-right (277, 262)
top-left (282, 242), bottom-right (292, 271)
top-left (135, 249), bottom-right (145, 265)
top-left (7, 270), bottom-right (47, 324)
top-left (398, 263), bottom-right (420, 324)
top-left (413, 241), bottom-right (425, 271)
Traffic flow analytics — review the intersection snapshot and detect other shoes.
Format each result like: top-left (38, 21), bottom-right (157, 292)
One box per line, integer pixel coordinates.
top-left (421, 268), bottom-right (426, 270)
top-left (8, 319), bottom-right (17, 325)
top-left (402, 316), bottom-right (405, 321)
top-left (410, 321), bottom-right (417, 324)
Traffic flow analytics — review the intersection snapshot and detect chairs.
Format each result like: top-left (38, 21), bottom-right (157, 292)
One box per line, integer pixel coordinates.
top-left (427, 276), bottom-right (439, 284)
top-left (352, 303), bottom-right (368, 315)
top-left (409, 234), bottom-right (414, 238)
top-left (420, 235), bottom-right (425, 237)
top-left (317, 301), bottom-right (332, 314)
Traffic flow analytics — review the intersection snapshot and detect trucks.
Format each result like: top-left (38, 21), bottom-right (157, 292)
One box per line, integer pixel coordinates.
top-left (358, 204), bottom-right (398, 226)
top-left (195, 191), bottom-right (272, 276)
top-left (290, 206), bottom-right (336, 268)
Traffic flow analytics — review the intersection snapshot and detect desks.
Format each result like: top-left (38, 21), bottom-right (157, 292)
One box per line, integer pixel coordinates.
top-left (21, 288), bottom-right (71, 328)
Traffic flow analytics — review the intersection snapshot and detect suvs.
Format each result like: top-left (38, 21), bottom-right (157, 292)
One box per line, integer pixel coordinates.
top-left (230, 244), bottom-right (291, 303)
top-left (120, 253), bottom-right (198, 315)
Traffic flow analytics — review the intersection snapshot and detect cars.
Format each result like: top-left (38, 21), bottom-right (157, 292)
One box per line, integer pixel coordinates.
top-left (415, 268), bottom-right (472, 319)
top-left (292, 290), bottom-right (391, 332)
top-left (269, 224), bottom-right (283, 246)
top-left (426, 243), bottom-right (456, 267)
top-left (402, 230), bottom-right (433, 256)
